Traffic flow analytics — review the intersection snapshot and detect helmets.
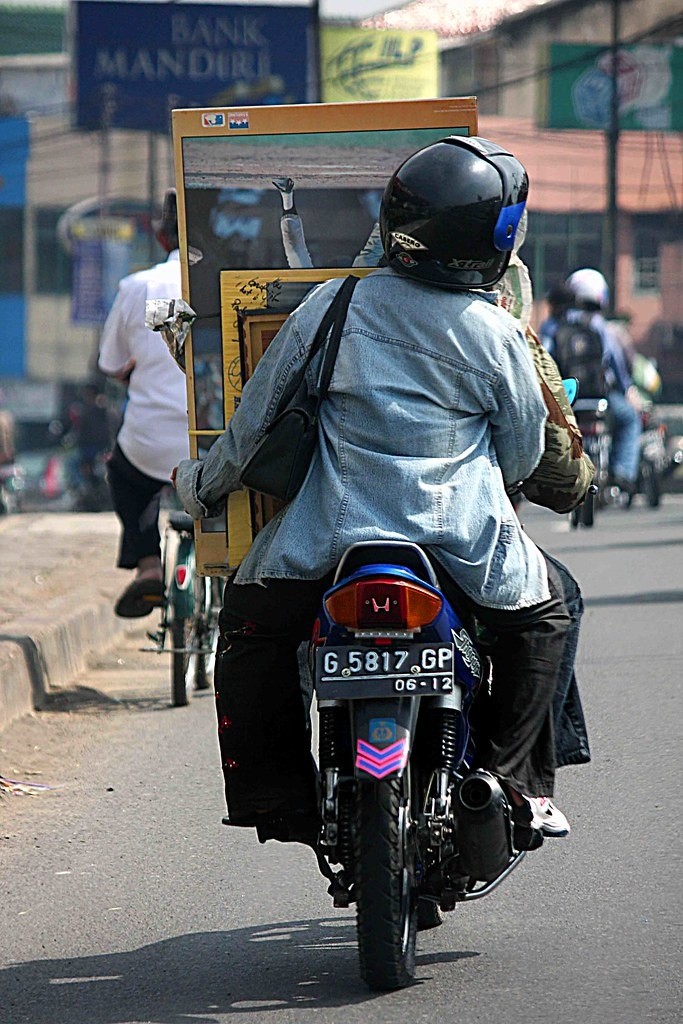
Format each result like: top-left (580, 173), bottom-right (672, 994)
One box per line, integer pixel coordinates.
top-left (563, 268), bottom-right (610, 313)
top-left (380, 136), bottom-right (529, 288)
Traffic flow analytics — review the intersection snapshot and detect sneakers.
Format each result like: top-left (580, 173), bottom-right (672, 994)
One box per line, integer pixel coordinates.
top-left (522, 793), bottom-right (571, 837)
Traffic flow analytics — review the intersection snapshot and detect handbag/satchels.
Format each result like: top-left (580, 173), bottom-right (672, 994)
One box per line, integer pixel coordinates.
top-left (238, 274), bottom-right (361, 503)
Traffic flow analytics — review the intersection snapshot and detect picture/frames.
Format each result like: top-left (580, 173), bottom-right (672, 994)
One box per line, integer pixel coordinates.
top-left (171, 94), bottom-right (479, 586)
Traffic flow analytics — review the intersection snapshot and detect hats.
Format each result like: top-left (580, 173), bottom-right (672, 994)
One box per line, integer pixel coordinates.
top-left (155, 188), bottom-right (178, 238)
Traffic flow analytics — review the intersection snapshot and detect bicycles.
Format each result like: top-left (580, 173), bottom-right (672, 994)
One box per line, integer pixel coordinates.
top-left (143, 491), bottom-right (223, 708)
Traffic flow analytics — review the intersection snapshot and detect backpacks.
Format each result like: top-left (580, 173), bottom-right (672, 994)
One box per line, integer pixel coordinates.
top-left (551, 310), bottom-right (608, 399)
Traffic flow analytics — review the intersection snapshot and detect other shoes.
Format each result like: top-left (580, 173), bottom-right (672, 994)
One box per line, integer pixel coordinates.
top-left (500, 779), bottom-right (534, 822)
top-left (222, 793), bottom-right (318, 828)
top-left (113, 561), bottom-right (164, 618)
top-left (612, 474), bottom-right (637, 494)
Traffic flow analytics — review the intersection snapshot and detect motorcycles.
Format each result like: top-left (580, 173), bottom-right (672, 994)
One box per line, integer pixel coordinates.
top-left (567, 394), bottom-right (670, 529)
top-left (167, 453), bottom-right (600, 993)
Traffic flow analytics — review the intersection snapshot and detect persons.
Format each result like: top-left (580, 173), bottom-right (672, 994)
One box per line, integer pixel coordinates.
top-left (64, 386), bottom-right (108, 512)
top-left (98, 188), bottom-right (191, 618)
top-left (170, 134), bottom-right (591, 837)
top-left (0, 388), bottom-right (16, 515)
top-left (538, 268), bottom-right (661, 493)
